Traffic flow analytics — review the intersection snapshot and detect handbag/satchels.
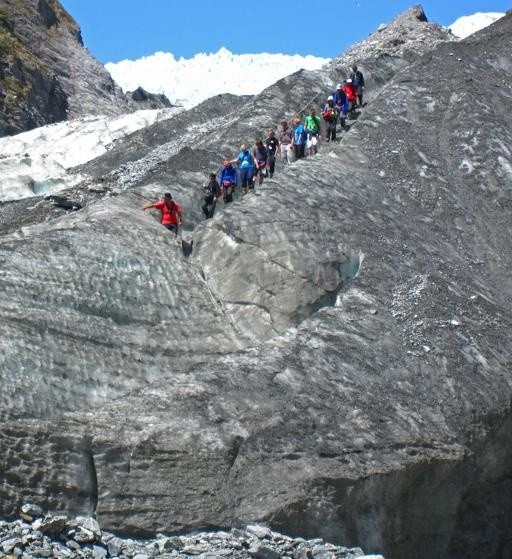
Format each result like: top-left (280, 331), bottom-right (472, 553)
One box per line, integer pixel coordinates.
top-left (203, 192), bottom-right (213, 204)
top-left (261, 168), bottom-right (268, 178)
top-left (323, 112), bottom-right (331, 119)
top-left (223, 180), bottom-right (231, 187)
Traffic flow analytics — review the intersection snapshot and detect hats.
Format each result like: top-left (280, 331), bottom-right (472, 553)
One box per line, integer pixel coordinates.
top-left (328, 96), bottom-right (334, 101)
top-left (347, 79), bottom-right (352, 83)
top-left (336, 84), bottom-right (342, 89)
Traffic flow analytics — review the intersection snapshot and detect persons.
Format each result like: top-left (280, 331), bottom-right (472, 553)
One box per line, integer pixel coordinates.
top-left (142, 193), bottom-right (183, 236)
top-left (199, 64), bottom-right (367, 220)
